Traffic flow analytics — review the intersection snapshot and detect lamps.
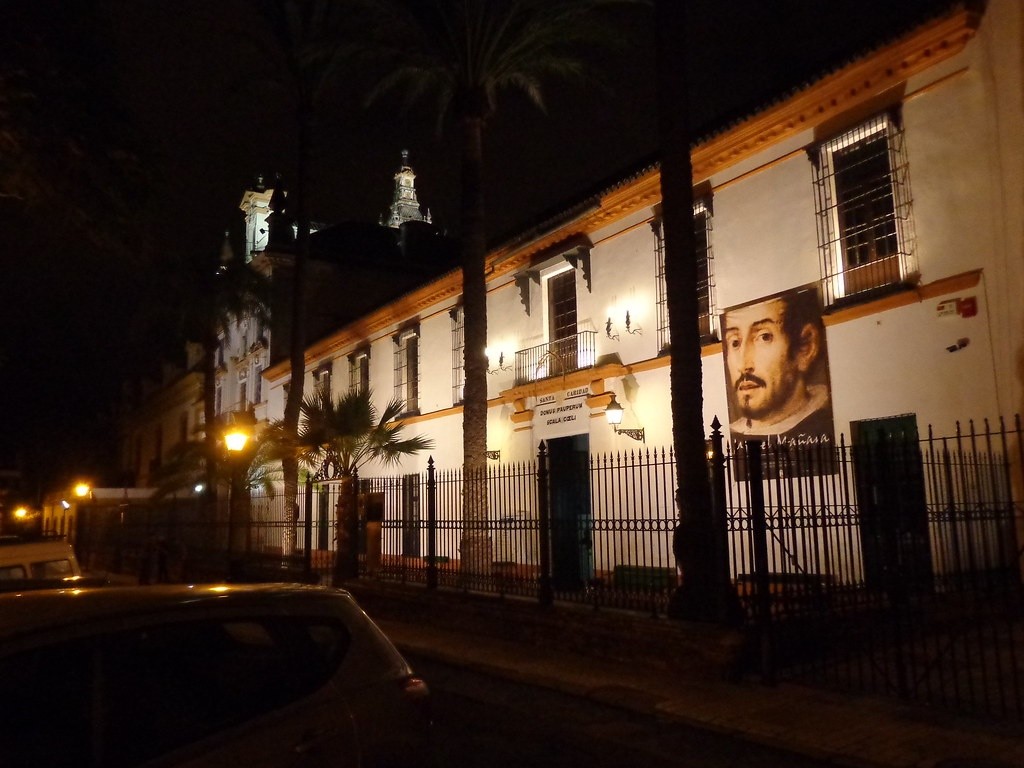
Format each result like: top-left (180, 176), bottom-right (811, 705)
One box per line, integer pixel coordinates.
top-left (498, 351), bottom-right (513, 371)
top-left (605, 308), bottom-right (620, 342)
top-left (625, 308), bottom-right (645, 335)
top-left (605, 392), bottom-right (644, 442)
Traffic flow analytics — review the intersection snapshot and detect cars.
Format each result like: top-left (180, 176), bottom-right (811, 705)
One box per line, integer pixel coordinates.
top-left (1, 584), bottom-right (469, 768)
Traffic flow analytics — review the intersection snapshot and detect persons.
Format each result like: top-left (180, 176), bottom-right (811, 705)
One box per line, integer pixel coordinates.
top-left (724, 286), bottom-right (835, 445)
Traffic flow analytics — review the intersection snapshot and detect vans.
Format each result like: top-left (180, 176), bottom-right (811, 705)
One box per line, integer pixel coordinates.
top-left (0, 536), bottom-right (82, 591)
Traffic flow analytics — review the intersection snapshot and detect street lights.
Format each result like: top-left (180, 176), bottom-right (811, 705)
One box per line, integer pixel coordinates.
top-left (224, 424), bottom-right (249, 554)
